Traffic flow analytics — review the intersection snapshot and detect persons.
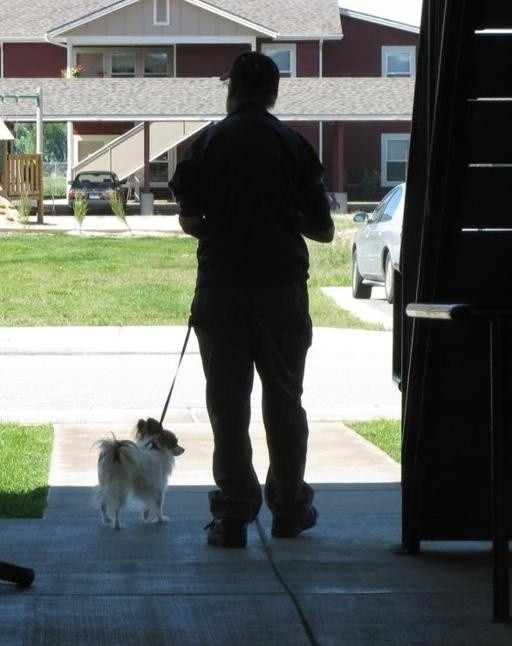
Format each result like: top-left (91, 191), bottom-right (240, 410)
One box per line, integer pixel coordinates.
top-left (167, 50), bottom-right (335, 549)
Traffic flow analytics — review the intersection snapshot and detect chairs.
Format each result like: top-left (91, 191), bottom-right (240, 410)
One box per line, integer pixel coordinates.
top-left (103, 179), bottom-right (112, 183)
top-left (82, 180), bottom-right (90, 183)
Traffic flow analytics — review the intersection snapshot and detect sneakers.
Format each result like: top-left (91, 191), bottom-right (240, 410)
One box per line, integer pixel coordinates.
top-left (272, 505), bottom-right (318, 536)
top-left (203, 518), bottom-right (249, 549)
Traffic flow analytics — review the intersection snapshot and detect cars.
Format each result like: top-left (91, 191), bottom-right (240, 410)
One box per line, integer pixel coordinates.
top-left (65, 169), bottom-right (129, 214)
top-left (348, 181), bottom-right (409, 304)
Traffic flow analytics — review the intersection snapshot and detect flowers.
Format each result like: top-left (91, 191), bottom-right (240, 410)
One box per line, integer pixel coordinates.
top-left (61, 63), bottom-right (86, 76)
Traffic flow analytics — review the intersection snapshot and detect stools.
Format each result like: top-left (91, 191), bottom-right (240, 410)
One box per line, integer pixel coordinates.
top-left (392, 303), bottom-right (510, 623)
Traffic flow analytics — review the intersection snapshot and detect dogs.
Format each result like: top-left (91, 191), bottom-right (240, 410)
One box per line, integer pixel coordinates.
top-left (90, 418), bottom-right (185, 531)
